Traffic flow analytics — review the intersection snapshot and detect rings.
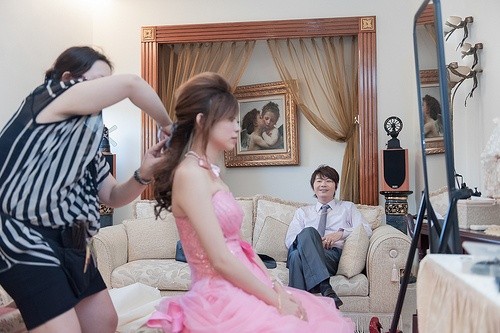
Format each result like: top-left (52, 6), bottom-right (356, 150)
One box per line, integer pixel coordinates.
top-left (328, 241), bottom-right (330, 242)
top-left (331, 242), bottom-right (333, 244)
top-left (301, 315), bottom-right (304, 319)
top-left (325, 240), bottom-right (327, 242)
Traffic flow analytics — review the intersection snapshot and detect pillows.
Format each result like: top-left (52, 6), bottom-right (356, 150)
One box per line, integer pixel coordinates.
top-left (337, 223), bottom-right (370, 279)
top-left (254, 215), bottom-right (290, 262)
top-left (122, 214), bottom-right (180, 262)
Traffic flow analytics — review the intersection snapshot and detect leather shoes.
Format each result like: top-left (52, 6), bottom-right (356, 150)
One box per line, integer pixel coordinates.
top-left (322, 288), bottom-right (343, 307)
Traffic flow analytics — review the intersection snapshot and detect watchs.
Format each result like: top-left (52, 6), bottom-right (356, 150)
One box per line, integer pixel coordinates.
top-left (135, 170), bottom-right (152, 184)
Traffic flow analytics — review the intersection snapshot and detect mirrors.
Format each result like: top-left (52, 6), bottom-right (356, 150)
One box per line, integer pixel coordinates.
top-left (413, 0), bottom-right (462, 254)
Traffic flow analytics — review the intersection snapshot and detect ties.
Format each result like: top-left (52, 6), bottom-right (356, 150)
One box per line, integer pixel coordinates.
top-left (317, 205), bottom-right (330, 238)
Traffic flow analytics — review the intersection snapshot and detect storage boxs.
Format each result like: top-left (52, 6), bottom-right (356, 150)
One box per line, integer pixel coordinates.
top-left (456, 199), bottom-right (500, 229)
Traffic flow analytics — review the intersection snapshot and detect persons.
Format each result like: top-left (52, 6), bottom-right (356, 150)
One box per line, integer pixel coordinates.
top-left (285, 166), bottom-right (372, 306)
top-left (0, 46), bottom-right (173, 333)
top-left (145, 74), bottom-right (359, 333)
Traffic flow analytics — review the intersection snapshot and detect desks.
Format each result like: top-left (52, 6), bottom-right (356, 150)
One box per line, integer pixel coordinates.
top-left (417, 224), bottom-right (500, 252)
top-left (415, 255), bottom-right (500, 333)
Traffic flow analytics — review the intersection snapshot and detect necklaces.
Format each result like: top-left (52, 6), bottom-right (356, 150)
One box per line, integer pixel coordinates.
top-left (188, 151), bottom-right (208, 168)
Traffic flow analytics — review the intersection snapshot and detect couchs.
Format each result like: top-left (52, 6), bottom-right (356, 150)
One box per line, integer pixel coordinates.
top-left (91, 195), bottom-right (411, 333)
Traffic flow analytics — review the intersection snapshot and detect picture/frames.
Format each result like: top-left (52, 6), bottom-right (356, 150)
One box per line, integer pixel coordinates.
top-left (224, 79), bottom-right (299, 168)
top-left (419, 69), bottom-right (445, 154)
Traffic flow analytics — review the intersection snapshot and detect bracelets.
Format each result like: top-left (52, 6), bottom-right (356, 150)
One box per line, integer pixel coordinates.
top-left (273, 279), bottom-right (277, 282)
